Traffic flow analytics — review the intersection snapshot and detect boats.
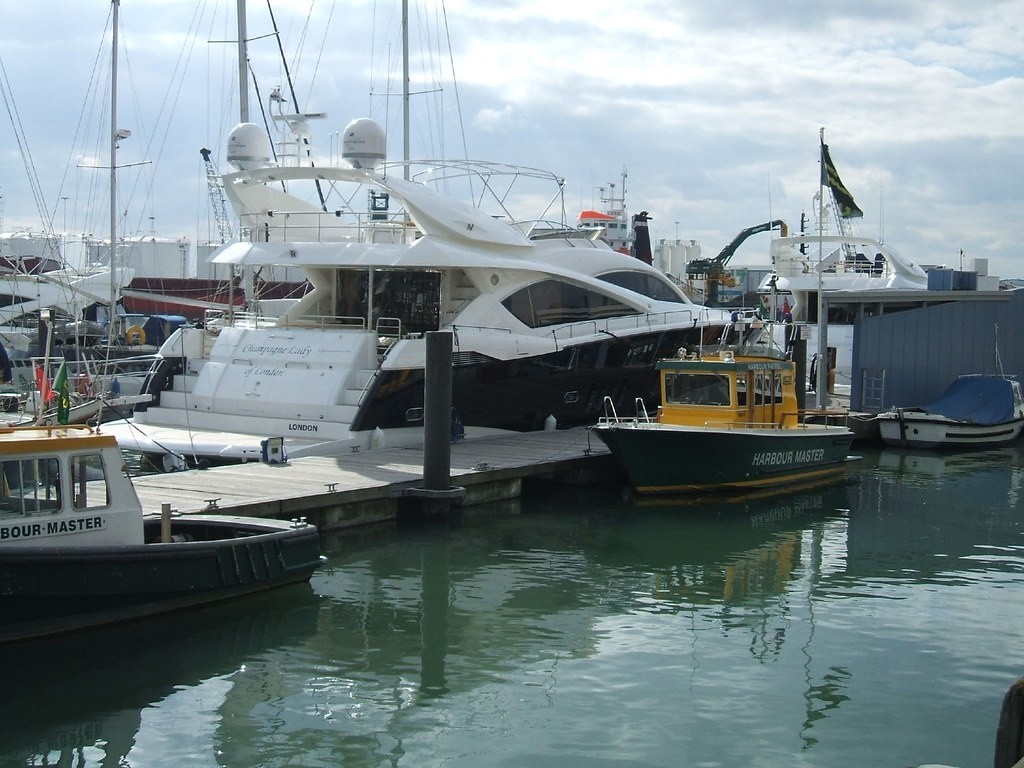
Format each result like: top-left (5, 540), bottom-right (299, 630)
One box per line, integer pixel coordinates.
top-left (0, 0), bottom-right (1024, 644)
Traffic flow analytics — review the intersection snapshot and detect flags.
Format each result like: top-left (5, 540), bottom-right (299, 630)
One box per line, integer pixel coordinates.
top-left (784, 297), bottom-right (790, 319)
top-left (822, 142), bottom-right (863, 219)
top-left (51, 360), bottom-right (69, 425)
top-left (35, 367), bottom-right (55, 404)
top-left (760, 298), bottom-right (766, 317)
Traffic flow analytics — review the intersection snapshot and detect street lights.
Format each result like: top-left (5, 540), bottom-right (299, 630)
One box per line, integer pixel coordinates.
top-left (61, 197), bottom-right (70, 263)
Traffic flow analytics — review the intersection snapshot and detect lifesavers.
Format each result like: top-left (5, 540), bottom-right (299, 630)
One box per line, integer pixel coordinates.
top-left (127, 325), bottom-right (146, 345)
